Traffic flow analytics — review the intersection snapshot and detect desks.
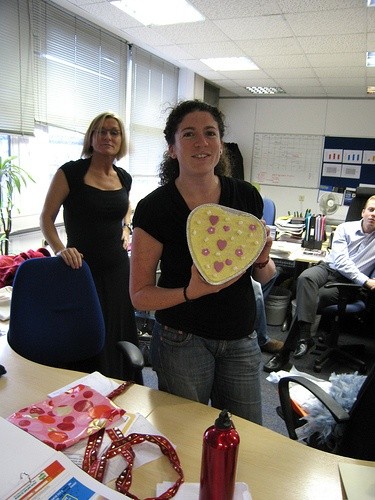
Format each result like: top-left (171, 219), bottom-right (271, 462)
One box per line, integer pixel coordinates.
top-left (1, 320), bottom-right (375, 500)
top-left (271, 240), bottom-right (326, 275)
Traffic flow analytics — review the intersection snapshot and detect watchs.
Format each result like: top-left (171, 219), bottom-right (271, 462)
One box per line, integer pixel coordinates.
top-left (124, 223), bottom-right (132, 235)
top-left (253, 256), bottom-right (269, 269)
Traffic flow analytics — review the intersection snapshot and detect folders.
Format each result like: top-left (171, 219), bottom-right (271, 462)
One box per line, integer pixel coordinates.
top-left (307, 214), bottom-right (316, 242)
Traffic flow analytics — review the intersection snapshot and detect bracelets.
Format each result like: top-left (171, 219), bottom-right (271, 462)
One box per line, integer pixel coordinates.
top-left (183, 286), bottom-right (192, 304)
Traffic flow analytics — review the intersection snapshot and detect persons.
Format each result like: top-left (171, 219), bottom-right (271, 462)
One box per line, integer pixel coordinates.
top-left (250, 275), bottom-right (284, 353)
top-left (262, 195), bottom-right (375, 373)
top-left (39, 112), bottom-right (144, 386)
top-left (129, 99), bottom-right (278, 427)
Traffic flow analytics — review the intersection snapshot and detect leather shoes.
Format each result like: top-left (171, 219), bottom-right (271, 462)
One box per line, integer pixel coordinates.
top-left (263, 353), bottom-right (292, 372)
top-left (293, 335), bottom-right (314, 359)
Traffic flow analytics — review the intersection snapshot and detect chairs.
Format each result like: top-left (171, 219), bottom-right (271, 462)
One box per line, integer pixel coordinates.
top-left (275, 368), bottom-right (375, 462)
top-left (7, 256), bottom-right (145, 379)
top-left (310, 270), bottom-right (375, 373)
top-left (262, 199), bottom-right (276, 225)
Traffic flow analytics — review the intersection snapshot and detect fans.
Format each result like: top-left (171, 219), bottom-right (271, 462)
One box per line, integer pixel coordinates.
top-left (318, 192), bottom-right (339, 215)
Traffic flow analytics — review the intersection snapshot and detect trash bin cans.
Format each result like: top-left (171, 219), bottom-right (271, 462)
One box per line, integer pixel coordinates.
top-left (262, 286), bottom-right (292, 326)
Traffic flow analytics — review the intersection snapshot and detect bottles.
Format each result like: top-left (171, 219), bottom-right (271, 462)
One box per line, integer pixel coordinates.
top-left (199, 410), bottom-right (240, 500)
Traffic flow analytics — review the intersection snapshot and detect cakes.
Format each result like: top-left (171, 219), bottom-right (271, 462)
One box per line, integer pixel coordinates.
top-left (186, 203), bottom-right (267, 286)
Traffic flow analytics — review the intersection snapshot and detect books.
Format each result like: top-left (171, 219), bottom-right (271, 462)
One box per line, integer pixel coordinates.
top-left (291, 217), bottom-right (305, 224)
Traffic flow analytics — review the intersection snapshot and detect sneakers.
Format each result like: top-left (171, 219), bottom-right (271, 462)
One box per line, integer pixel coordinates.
top-left (261, 338), bottom-right (285, 352)
